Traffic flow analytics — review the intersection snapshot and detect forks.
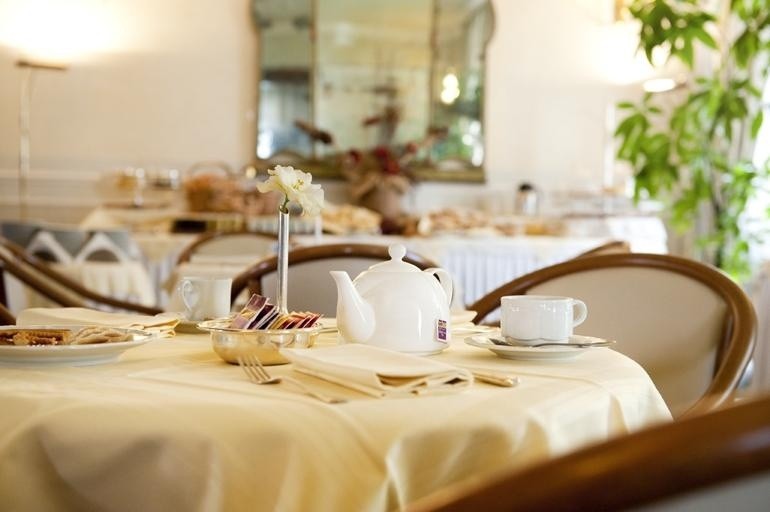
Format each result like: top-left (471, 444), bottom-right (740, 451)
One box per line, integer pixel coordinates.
top-left (233, 352), bottom-right (351, 408)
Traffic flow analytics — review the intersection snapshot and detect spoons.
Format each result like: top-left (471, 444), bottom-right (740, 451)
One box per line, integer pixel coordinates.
top-left (489, 336), bottom-right (619, 353)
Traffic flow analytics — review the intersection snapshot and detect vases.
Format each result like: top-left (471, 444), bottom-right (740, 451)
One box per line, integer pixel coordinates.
top-left (276, 203), bottom-right (288, 318)
top-left (352, 175), bottom-right (406, 223)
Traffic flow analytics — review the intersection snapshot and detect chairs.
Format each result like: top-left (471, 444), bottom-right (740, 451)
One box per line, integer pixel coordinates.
top-left (432, 391), bottom-right (769, 511)
top-left (573, 240), bottom-right (632, 260)
top-left (466, 252), bottom-right (758, 422)
top-left (1, 235), bottom-right (165, 325)
top-left (231, 243), bottom-right (454, 318)
top-left (163, 230), bottom-right (302, 296)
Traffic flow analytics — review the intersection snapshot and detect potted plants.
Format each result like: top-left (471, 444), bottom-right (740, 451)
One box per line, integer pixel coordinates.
top-left (612, 1), bottom-right (769, 387)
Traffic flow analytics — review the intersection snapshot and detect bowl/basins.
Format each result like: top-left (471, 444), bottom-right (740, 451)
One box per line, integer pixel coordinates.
top-left (195, 310), bottom-right (324, 368)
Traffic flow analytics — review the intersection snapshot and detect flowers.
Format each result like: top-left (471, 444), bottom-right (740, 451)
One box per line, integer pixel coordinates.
top-left (292, 80), bottom-right (447, 172)
top-left (255, 164), bottom-right (324, 217)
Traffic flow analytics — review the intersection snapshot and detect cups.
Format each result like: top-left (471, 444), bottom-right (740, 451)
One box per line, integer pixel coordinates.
top-left (498, 292), bottom-right (588, 347)
top-left (179, 276), bottom-right (233, 320)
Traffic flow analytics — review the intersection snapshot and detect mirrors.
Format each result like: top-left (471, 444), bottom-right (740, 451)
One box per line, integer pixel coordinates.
top-left (248, 1), bottom-right (496, 184)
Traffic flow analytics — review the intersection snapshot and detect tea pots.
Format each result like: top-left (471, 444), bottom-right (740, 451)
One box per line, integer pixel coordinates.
top-left (328, 241), bottom-right (456, 360)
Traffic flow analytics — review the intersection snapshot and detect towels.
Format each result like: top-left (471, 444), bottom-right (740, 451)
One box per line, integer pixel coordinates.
top-left (15, 307), bottom-right (180, 339)
top-left (278, 343), bottom-right (474, 400)
top-left (450, 310), bottom-right (478, 335)
top-left (316, 317), bottom-right (339, 333)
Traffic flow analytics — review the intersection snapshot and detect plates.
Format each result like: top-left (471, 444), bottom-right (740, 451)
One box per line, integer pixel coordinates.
top-left (0, 321), bottom-right (160, 369)
top-left (464, 328), bottom-right (619, 363)
top-left (154, 312), bottom-right (244, 334)
